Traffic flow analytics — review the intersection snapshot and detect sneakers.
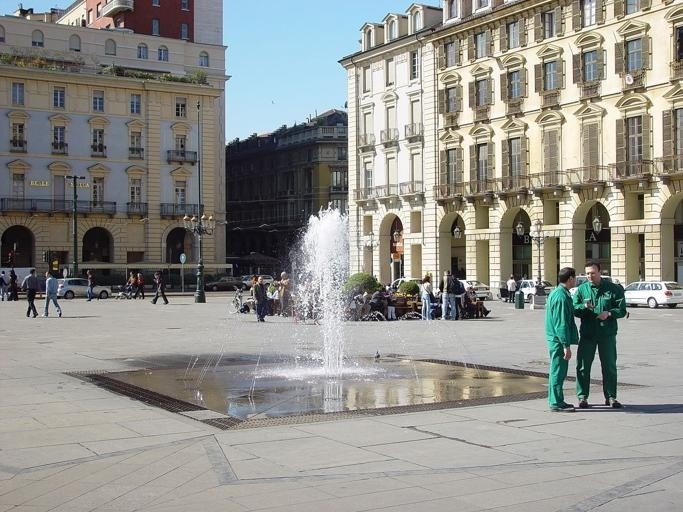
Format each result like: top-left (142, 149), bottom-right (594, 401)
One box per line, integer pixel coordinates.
top-left (58, 313), bottom-right (61, 317)
top-left (552, 399), bottom-right (621, 412)
top-left (132, 296), bottom-right (136, 298)
top-left (98, 295), bottom-right (100, 300)
top-left (87, 299), bottom-right (91, 301)
top-left (150, 300), bottom-right (155, 303)
top-left (441, 318), bottom-right (446, 320)
top-left (422, 317), bottom-right (426, 320)
top-left (143, 296), bottom-right (144, 299)
top-left (163, 302), bottom-right (168, 304)
top-left (42, 314), bottom-right (47, 317)
top-left (262, 319), bottom-right (265, 321)
top-left (258, 319), bottom-right (261, 322)
top-left (26, 314), bottom-right (29, 317)
top-left (2, 298), bottom-right (18, 301)
top-left (32, 313), bottom-right (38, 318)
top-left (427, 318), bottom-right (432, 320)
top-left (451, 318), bottom-right (457, 321)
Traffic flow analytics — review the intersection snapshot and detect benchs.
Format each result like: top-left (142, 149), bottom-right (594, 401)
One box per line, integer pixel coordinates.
top-left (469, 300), bottom-right (484, 317)
top-left (391, 299), bottom-right (418, 318)
top-left (529, 294), bottom-right (547, 309)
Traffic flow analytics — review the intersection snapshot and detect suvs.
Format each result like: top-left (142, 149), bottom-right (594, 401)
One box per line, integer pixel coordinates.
top-left (237, 274), bottom-right (273, 290)
top-left (622, 280), bottom-right (682, 309)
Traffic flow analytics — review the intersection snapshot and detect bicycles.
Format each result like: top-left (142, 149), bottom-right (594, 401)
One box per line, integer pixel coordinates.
top-left (226, 285), bottom-right (244, 315)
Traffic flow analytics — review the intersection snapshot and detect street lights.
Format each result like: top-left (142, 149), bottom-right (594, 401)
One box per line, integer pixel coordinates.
top-left (63, 174), bottom-right (87, 277)
top-left (527, 216), bottom-right (548, 310)
top-left (181, 211), bottom-right (216, 305)
top-left (367, 230), bottom-right (375, 278)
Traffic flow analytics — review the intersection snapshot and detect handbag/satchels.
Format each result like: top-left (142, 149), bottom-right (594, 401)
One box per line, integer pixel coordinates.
top-left (430, 292), bottom-right (436, 302)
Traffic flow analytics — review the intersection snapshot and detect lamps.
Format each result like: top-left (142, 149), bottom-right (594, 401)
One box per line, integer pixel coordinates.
top-left (452, 216), bottom-right (462, 240)
top-left (513, 209), bottom-right (525, 239)
top-left (392, 218), bottom-right (401, 243)
top-left (591, 202), bottom-right (603, 237)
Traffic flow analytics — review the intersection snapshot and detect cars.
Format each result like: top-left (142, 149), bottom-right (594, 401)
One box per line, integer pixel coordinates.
top-left (567, 275), bottom-right (621, 297)
top-left (495, 278), bottom-right (551, 304)
top-left (434, 278), bottom-right (490, 301)
top-left (204, 273), bottom-right (244, 293)
top-left (54, 277), bottom-right (113, 300)
top-left (384, 277), bottom-right (423, 292)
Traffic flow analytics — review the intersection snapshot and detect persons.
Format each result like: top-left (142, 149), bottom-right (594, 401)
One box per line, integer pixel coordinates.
top-left (507, 277), bottom-right (517, 303)
top-left (42, 270), bottom-right (62, 317)
top-left (543, 268), bottom-right (580, 411)
top-left (21, 268), bottom-right (42, 317)
top-left (240, 268), bottom-right (492, 321)
top-left (150, 272), bottom-right (169, 305)
top-left (0, 266), bottom-right (155, 301)
top-left (572, 261), bottom-right (627, 409)
